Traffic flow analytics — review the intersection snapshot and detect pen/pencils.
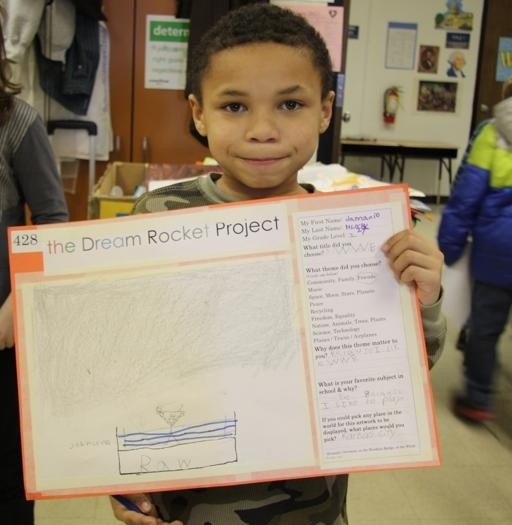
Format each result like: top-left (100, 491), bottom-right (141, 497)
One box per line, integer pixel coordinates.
top-left (113, 496), bottom-right (148, 515)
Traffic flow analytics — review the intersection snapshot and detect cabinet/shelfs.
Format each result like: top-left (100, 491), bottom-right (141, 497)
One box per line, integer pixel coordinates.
top-left (58, 1), bottom-right (211, 222)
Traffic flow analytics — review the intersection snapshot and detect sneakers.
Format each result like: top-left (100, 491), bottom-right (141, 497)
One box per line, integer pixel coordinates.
top-left (449, 398), bottom-right (494, 423)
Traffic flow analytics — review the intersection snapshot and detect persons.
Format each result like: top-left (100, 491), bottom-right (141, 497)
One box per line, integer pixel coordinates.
top-left (0, 14), bottom-right (73, 525)
top-left (437, 76), bottom-right (512, 422)
top-left (104, 1), bottom-right (449, 525)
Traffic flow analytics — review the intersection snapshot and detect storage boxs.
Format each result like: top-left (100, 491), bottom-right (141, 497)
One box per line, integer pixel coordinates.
top-left (87, 161), bottom-right (149, 219)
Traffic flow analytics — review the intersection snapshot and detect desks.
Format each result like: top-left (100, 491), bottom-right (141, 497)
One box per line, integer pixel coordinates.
top-left (339, 134), bottom-right (456, 205)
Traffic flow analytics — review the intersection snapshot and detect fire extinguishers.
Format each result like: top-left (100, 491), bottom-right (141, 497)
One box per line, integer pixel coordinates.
top-left (384, 85), bottom-right (404, 123)
